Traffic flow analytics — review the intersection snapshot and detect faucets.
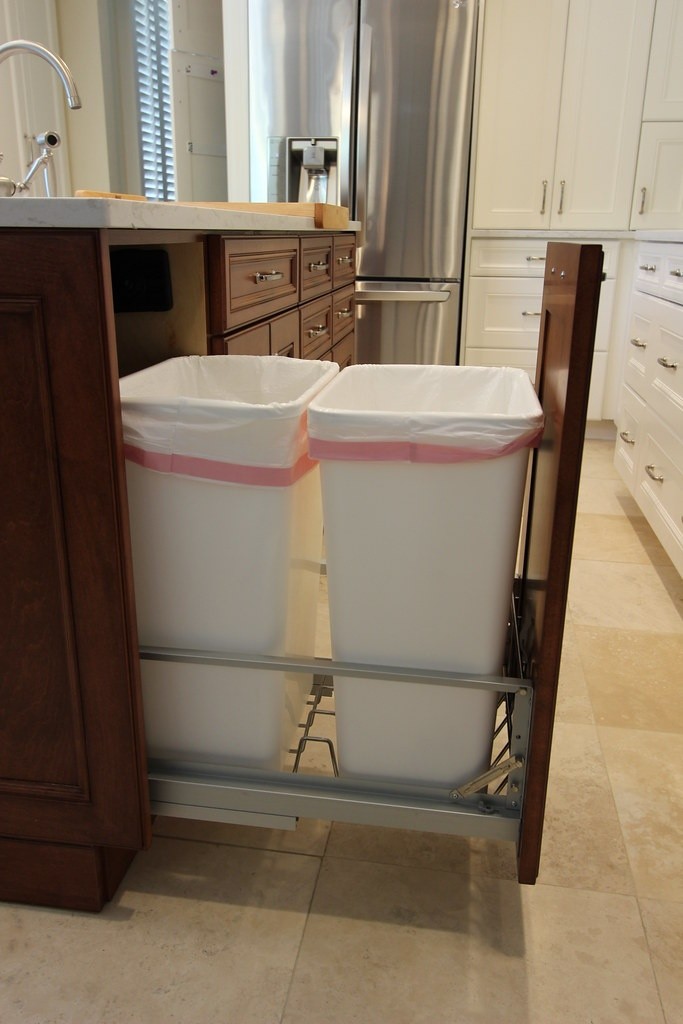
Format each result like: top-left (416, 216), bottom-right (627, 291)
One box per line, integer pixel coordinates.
top-left (0, 35), bottom-right (84, 112)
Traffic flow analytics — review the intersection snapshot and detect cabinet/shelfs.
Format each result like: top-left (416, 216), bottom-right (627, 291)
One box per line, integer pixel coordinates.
top-left (1, 0), bottom-right (682, 913)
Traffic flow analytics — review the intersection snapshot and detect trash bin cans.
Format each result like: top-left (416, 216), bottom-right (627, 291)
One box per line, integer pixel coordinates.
top-left (305, 363), bottom-right (544, 794)
top-left (118, 354), bottom-right (340, 763)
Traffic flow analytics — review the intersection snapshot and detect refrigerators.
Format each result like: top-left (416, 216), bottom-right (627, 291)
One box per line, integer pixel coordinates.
top-left (249, 0), bottom-right (481, 366)
